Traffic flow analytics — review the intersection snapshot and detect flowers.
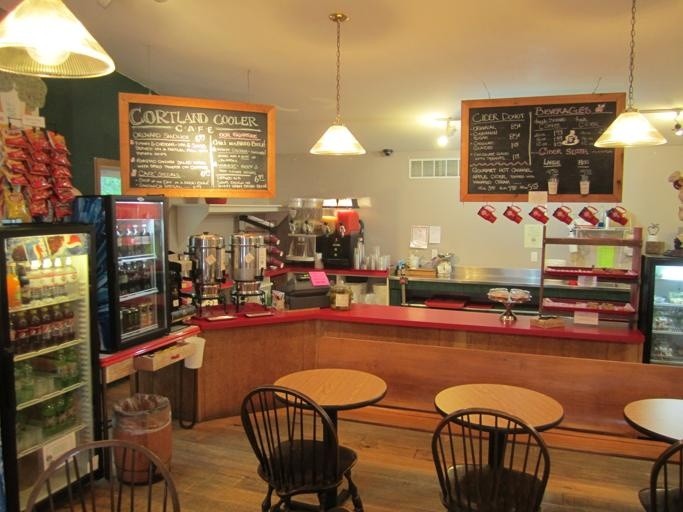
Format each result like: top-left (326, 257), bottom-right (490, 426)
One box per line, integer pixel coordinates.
top-left (431, 249), bottom-right (455, 265)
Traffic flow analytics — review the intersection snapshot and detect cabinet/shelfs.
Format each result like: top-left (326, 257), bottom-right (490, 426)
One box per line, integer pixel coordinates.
top-left (537, 225), bottom-right (643, 330)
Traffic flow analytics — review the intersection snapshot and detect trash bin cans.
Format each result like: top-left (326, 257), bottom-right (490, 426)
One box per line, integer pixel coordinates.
top-left (113, 393), bottom-right (173, 486)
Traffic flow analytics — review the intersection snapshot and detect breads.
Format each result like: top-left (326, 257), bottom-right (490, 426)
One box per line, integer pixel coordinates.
top-left (586, 302), bottom-right (598, 309)
top-left (601, 302), bottom-right (614, 311)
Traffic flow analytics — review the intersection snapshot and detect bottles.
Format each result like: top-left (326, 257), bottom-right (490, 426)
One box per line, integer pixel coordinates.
top-left (329, 280), bottom-right (351, 310)
top-left (395, 266), bottom-right (401, 276)
top-left (401, 264), bottom-right (405, 276)
top-left (8, 258), bottom-right (81, 438)
top-left (260, 269), bottom-right (272, 306)
top-left (116, 223), bottom-right (155, 334)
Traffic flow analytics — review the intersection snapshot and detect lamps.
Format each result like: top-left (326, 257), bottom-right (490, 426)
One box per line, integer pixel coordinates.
top-left (309, 13), bottom-right (366, 155)
top-left (594, 0), bottom-right (667, 148)
top-left (435, 127), bottom-right (457, 147)
top-left (672, 109), bottom-right (683, 137)
top-left (0, 0), bottom-right (117, 79)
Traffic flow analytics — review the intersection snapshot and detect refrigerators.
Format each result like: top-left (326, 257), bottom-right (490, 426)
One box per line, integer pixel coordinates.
top-left (72, 195), bottom-right (171, 354)
top-left (0, 222), bottom-right (102, 512)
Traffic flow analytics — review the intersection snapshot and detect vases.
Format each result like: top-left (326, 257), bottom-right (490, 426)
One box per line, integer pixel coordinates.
top-left (437, 260), bottom-right (452, 273)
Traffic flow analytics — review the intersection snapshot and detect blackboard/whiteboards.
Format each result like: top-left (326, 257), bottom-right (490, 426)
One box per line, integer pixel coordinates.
top-left (460, 92), bottom-right (626, 202)
top-left (118, 91), bottom-right (276, 198)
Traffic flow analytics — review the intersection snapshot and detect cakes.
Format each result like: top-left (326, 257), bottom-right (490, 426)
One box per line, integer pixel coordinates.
top-left (489, 291), bottom-right (508, 300)
top-left (511, 292), bottom-right (530, 302)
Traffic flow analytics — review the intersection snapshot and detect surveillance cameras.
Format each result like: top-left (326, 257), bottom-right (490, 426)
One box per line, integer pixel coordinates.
top-left (383, 149), bottom-right (393, 156)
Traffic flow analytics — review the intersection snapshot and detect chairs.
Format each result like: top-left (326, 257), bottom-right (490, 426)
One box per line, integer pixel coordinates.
top-left (638, 440), bottom-right (683, 512)
top-left (26, 440), bottom-right (180, 512)
top-left (432, 408), bottom-right (552, 512)
top-left (240, 385), bottom-right (363, 512)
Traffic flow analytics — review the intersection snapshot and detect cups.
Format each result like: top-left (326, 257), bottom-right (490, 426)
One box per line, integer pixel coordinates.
top-left (354, 242), bottom-right (390, 271)
top-left (579, 181), bottom-right (590, 195)
top-left (548, 181), bottom-right (558, 194)
top-left (477, 204), bottom-right (628, 226)
top-left (184, 337), bottom-right (206, 369)
top-left (314, 252), bottom-right (324, 269)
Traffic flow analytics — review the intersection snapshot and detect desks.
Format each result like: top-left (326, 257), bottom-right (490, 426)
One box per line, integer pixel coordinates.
top-left (98, 325), bottom-right (206, 480)
